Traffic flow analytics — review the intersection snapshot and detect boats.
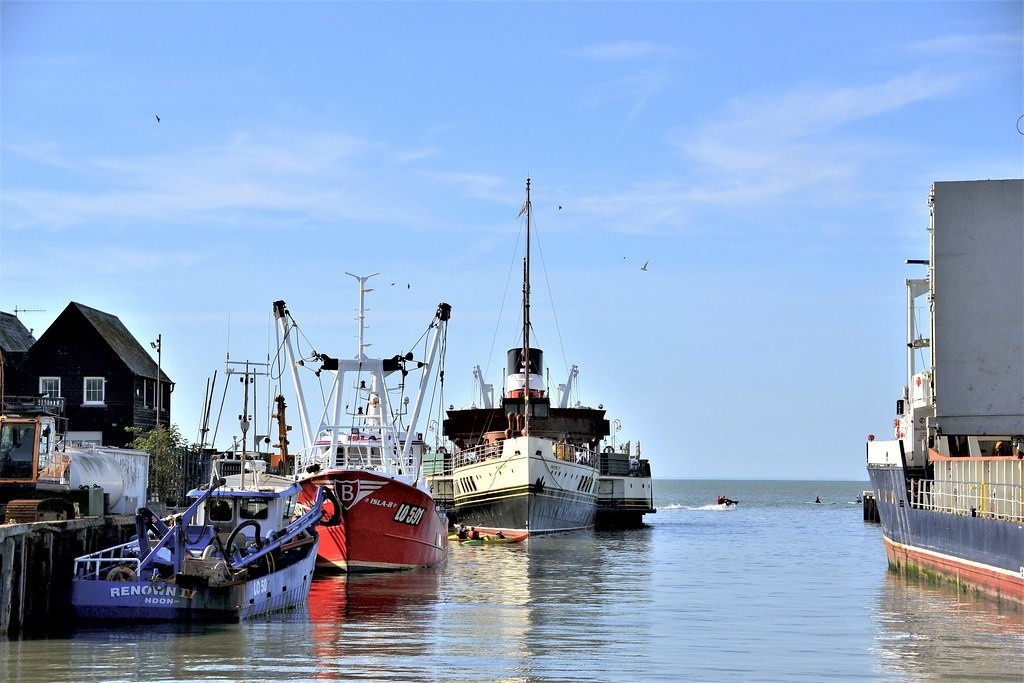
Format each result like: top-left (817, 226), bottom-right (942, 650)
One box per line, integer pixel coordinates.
top-left (805, 501), bottom-right (836, 505)
top-left (268, 271), bottom-right (449, 577)
top-left (71, 313), bottom-right (325, 628)
top-left (867, 180), bottom-right (1023, 608)
top-left (595, 418), bottom-right (659, 527)
top-left (448, 530), bottom-right (494, 541)
top-left (461, 532), bottom-right (530, 549)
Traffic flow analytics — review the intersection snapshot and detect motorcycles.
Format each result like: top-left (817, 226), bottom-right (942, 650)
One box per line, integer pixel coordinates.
top-left (716, 495), bottom-right (739, 508)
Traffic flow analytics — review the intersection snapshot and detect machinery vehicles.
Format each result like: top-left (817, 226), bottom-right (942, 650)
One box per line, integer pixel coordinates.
top-left (0, 415), bottom-right (76, 525)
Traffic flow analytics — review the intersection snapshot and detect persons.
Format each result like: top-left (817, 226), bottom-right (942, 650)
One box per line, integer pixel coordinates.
top-left (816, 495), bottom-right (821, 504)
top-left (856, 494), bottom-right (862, 504)
top-left (454, 437), bottom-right (503, 465)
top-left (717, 495), bottom-right (727, 506)
top-left (457, 523), bottom-right (506, 541)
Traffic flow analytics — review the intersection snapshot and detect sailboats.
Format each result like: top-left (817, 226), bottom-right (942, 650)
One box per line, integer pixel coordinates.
top-left (424, 178), bottom-right (604, 544)
